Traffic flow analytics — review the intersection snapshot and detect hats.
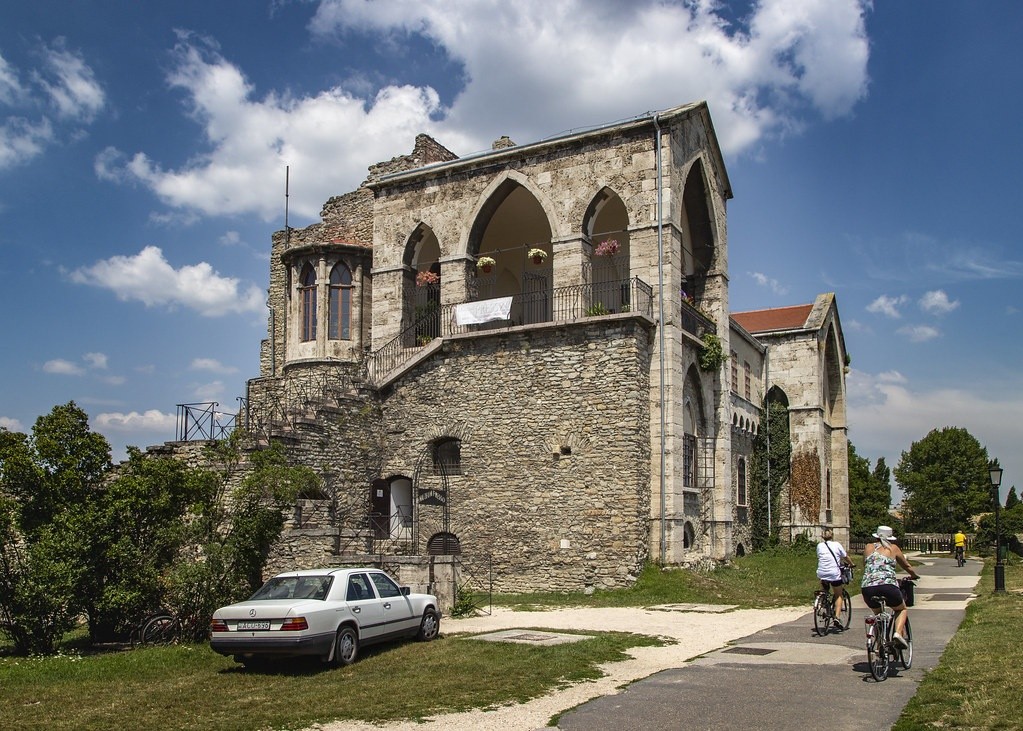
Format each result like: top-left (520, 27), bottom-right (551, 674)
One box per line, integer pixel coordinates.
top-left (872, 526), bottom-right (897, 551)
top-left (958, 530), bottom-right (962, 532)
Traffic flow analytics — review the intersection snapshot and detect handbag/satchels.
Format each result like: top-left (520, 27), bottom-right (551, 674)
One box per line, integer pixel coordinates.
top-left (842, 574), bottom-right (849, 585)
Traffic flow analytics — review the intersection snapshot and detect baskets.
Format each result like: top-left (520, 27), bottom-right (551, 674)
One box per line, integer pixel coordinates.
top-left (898, 579), bottom-right (916, 606)
top-left (844, 569), bottom-right (853, 581)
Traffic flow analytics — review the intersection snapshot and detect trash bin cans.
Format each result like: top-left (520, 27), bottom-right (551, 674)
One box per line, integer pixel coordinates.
top-left (938, 542), bottom-right (950, 551)
top-left (1000, 546), bottom-right (1007, 559)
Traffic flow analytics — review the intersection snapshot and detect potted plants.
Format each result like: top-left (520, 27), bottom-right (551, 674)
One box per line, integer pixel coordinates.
top-left (527, 248), bottom-right (549, 265)
top-left (416, 334), bottom-right (432, 348)
top-left (475, 256), bottom-right (497, 273)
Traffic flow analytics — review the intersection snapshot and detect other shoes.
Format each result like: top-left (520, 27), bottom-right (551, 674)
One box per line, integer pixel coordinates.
top-left (893, 632), bottom-right (909, 649)
top-left (955, 555), bottom-right (958, 558)
top-left (833, 618), bottom-right (843, 629)
top-left (962, 559), bottom-right (966, 563)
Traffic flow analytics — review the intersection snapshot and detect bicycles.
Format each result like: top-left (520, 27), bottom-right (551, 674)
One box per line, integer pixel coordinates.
top-left (812, 564), bottom-right (859, 637)
top-left (141, 593), bottom-right (207, 649)
top-left (863, 576), bottom-right (923, 682)
top-left (957, 551), bottom-right (966, 567)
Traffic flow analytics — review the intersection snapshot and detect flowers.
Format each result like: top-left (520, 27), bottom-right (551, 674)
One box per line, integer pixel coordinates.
top-left (595, 239), bottom-right (622, 257)
top-left (415, 271), bottom-right (439, 287)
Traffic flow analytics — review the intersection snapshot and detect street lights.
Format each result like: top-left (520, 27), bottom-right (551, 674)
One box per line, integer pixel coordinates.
top-left (988, 460), bottom-right (1006, 593)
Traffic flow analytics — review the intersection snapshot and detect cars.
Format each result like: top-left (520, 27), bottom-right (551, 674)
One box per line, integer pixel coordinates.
top-left (210, 568), bottom-right (443, 666)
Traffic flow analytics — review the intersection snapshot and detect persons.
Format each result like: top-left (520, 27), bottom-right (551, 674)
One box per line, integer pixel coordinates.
top-left (861, 526), bottom-right (920, 668)
top-left (954, 529), bottom-right (966, 563)
top-left (815, 529), bottom-right (855, 630)
